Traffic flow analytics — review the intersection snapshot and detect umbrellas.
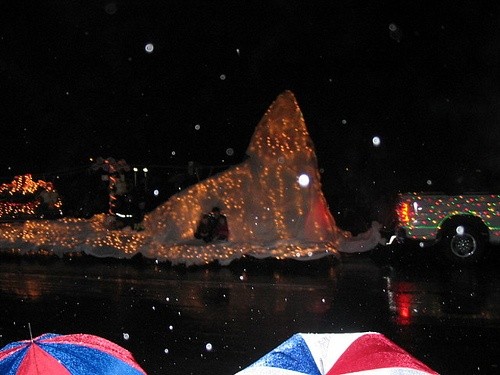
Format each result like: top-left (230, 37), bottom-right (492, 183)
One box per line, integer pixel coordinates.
top-left (235, 332), bottom-right (440, 375)
top-left (0, 322), bottom-right (147, 375)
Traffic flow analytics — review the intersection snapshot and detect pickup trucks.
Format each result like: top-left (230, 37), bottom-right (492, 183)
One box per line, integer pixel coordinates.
top-left (395, 193), bottom-right (500, 264)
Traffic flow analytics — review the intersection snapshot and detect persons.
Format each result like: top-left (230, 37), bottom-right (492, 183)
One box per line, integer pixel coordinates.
top-left (211, 207), bottom-right (229, 243)
top-left (194, 215), bottom-right (213, 243)
